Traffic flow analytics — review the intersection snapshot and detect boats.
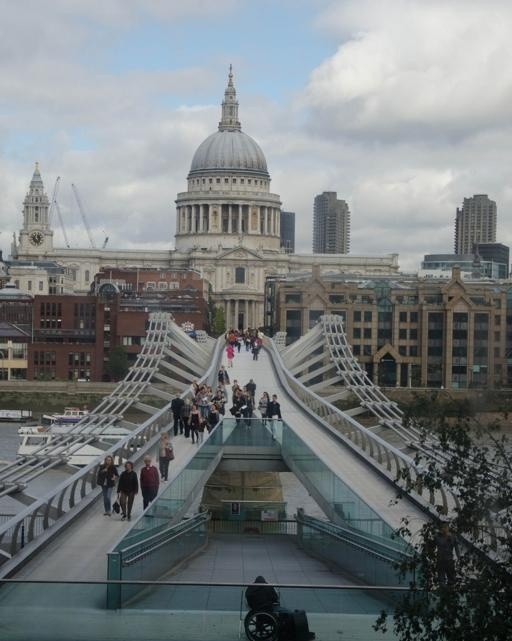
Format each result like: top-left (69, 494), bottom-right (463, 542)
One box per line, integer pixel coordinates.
top-left (0, 407), bottom-right (151, 468)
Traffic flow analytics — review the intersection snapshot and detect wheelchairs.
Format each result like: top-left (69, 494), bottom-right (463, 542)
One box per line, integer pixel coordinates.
top-left (241, 591), bottom-right (287, 640)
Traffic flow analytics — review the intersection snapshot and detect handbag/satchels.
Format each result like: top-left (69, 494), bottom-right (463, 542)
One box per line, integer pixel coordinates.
top-left (166, 447), bottom-right (174, 460)
top-left (113, 501), bottom-right (121, 513)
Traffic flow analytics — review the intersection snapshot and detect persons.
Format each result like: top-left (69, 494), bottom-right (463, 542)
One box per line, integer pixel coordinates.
top-left (258, 392), bottom-right (282, 442)
top-left (221, 328), bottom-right (262, 360)
top-left (217, 364), bottom-right (230, 386)
top-left (225, 343), bottom-right (235, 368)
top-left (229, 378), bottom-right (257, 429)
top-left (96, 455), bottom-right (119, 518)
top-left (244, 575), bottom-right (316, 641)
top-left (170, 380), bottom-right (226, 444)
top-left (117, 462), bottom-right (138, 522)
top-left (139, 455), bottom-right (159, 511)
top-left (158, 432), bottom-right (174, 482)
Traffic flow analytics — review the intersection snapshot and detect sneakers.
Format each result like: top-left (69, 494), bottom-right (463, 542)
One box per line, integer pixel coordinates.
top-left (161, 477), bottom-right (167, 484)
top-left (121, 515), bottom-right (131, 521)
